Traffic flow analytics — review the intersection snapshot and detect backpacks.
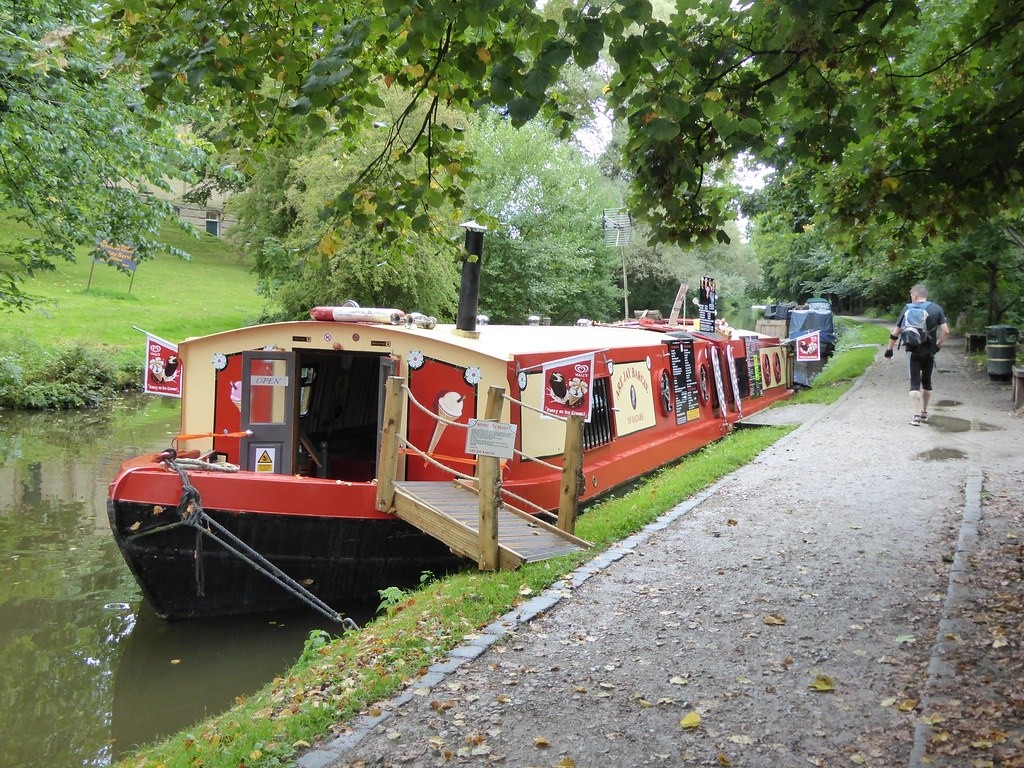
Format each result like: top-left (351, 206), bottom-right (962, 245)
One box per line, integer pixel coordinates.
top-left (899, 301), bottom-right (939, 348)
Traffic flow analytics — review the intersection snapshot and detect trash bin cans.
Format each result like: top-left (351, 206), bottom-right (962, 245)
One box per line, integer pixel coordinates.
top-left (985, 325), bottom-right (1018, 383)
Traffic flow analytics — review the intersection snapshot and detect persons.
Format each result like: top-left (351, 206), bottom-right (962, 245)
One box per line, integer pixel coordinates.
top-left (885, 285), bottom-right (949, 425)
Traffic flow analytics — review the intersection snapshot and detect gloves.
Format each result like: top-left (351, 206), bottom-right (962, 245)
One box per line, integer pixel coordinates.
top-left (936, 345), bottom-right (939, 352)
top-left (884, 349), bottom-right (893, 359)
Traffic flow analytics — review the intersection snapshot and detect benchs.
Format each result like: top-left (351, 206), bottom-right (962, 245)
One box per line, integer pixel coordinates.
top-left (1012, 363), bottom-right (1024, 409)
top-left (966, 333), bottom-right (986, 353)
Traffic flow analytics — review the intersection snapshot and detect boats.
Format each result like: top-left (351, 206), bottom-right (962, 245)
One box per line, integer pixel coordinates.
top-left (102, 208), bottom-right (802, 620)
top-left (754, 296), bottom-right (835, 358)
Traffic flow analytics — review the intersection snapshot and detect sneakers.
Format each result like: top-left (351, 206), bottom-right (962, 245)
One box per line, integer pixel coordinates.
top-left (908, 415), bottom-right (920, 426)
top-left (920, 410), bottom-right (928, 421)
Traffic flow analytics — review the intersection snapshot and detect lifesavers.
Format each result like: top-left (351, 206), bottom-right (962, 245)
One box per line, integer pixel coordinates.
top-left (310, 305), bottom-right (405, 326)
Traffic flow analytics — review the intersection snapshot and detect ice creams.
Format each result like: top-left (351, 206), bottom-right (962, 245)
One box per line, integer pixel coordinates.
top-left (149, 357), bottom-right (164, 382)
top-left (427, 391), bottom-right (466, 454)
top-left (230, 380), bottom-right (253, 423)
top-left (569, 377), bottom-right (588, 406)
top-left (701, 277), bottom-right (710, 298)
